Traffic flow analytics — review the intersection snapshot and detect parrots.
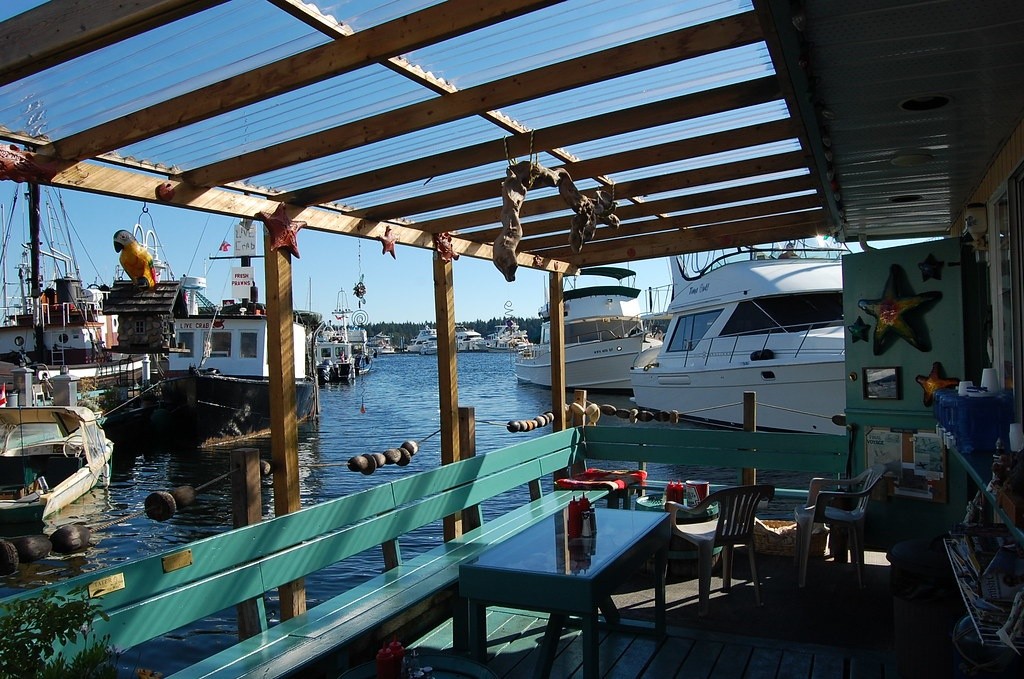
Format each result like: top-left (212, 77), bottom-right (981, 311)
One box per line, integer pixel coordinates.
top-left (113, 230), bottom-right (156, 291)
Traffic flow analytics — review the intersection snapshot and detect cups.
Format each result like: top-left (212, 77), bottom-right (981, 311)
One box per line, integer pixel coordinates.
top-left (958, 381), bottom-right (973, 396)
top-left (981, 368), bottom-right (999, 394)
top-left (1010, 423), bottom-right (1024, 452)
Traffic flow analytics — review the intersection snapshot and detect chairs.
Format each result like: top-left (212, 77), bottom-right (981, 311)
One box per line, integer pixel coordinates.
top-left (794, 463), bottom-right (889, 590)
top-left (666, 482), bottom-right (775, 617)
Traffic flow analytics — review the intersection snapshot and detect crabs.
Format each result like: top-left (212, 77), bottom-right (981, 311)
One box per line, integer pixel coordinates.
top-left (533, 255), bottom-right (544, 268)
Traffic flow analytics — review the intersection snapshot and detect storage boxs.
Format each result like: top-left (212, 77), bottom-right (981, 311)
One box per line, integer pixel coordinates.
top-left (1002, 490), bottom-right (1024, 529)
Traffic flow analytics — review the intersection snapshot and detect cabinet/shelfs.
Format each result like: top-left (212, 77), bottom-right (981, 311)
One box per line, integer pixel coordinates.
top-left (941, 441), bottom-right (1024, 650)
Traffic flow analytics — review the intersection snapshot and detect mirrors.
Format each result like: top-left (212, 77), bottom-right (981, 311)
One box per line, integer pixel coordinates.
top-left (862, 367), bottom-right (904, 400)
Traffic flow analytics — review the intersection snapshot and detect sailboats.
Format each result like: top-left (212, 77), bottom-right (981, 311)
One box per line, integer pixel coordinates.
top-left (2, 95), bottom-right (843, 585)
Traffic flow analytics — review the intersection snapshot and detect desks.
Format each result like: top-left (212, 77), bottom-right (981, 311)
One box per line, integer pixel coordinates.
top-left (460, 505), bottom-right (671, 679)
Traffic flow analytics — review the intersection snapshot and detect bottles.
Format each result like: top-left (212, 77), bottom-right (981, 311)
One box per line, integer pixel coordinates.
top-left (667, 479), bottom-right (675, 502)
top-left (376, 633), bottom-right (436, 679)
top-left (581, 511), bottom-right (592, 536)
top-left (675, 479), bottom-right (684, 504)
top-left (580, 492), bottom-right (590, 534)
top-left (590, 507), bottom-right (596, 535)
top-left (569, 496), bottom-right (580, 538)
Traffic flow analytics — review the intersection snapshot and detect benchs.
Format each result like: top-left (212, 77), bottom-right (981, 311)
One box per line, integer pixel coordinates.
top-left (0, 426), bottom-right (848, 679)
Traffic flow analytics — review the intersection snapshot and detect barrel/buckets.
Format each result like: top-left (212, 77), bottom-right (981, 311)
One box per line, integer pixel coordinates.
top-left (686, 480), bottom-right (710, 508)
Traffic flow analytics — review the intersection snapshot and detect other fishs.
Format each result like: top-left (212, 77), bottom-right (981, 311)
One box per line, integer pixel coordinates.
top-left (0, 145), bottom-right (58, 186)
top-left (433, 232), bottom-right (459, 263)
